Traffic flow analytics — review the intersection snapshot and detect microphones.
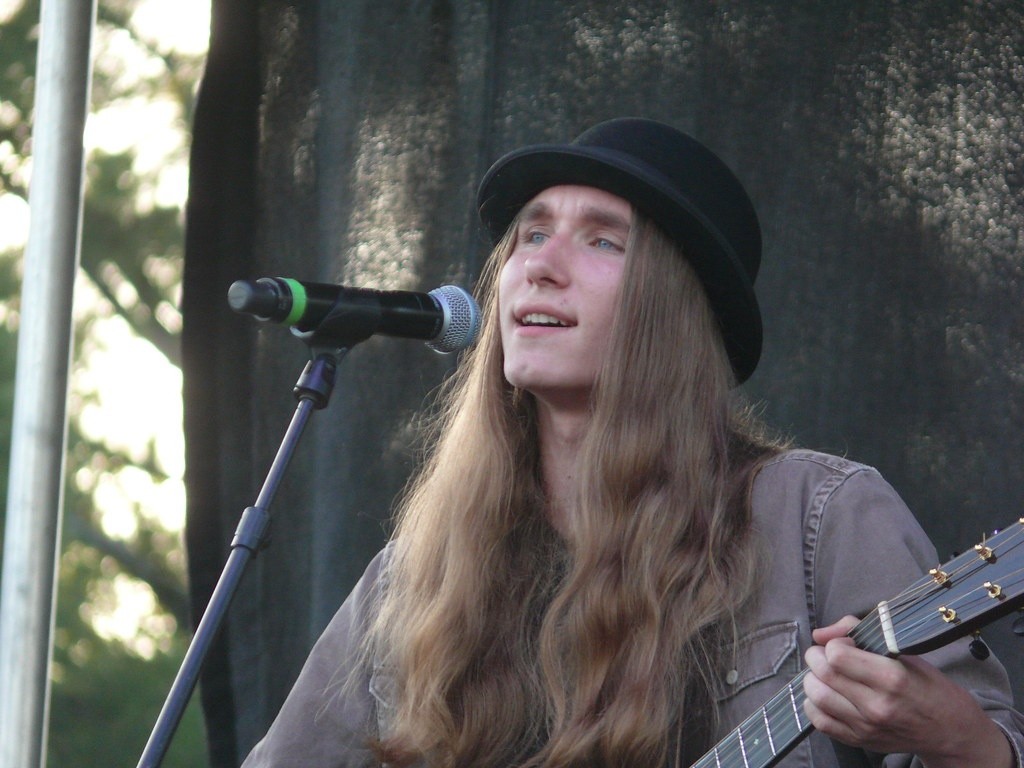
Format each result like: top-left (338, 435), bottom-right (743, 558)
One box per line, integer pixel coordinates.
top-left (228, 276), bottom-right (482, 354)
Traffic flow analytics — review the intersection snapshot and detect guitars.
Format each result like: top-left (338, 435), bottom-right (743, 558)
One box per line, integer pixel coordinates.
top-left (687, 512), bottom-right (1024, 767)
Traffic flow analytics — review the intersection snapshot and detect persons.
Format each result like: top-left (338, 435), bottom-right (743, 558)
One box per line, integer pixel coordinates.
top-left (239, 117), bottom-right (1024, 767)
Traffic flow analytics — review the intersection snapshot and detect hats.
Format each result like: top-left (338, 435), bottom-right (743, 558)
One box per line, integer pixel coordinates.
top-left (475, 117), bottom-right (765, 393)
